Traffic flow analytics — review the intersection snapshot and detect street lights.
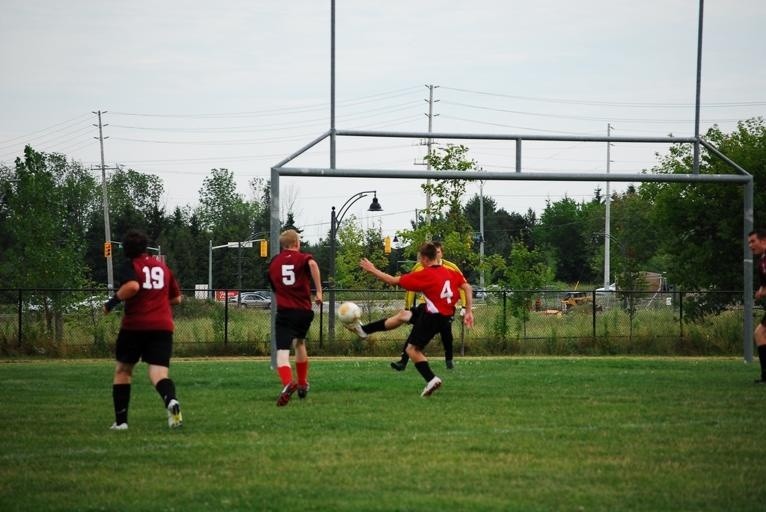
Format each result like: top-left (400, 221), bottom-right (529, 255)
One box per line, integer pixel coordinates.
top-left (328, 190), bottom-right (385, 341)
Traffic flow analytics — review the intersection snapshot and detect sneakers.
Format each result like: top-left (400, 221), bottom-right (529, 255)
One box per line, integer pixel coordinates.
top-left (346, 323), bottom-right (369, 338)
top-left (109, 422), bottom-right (128, 431)
top-left (167, 399), bottom-right (182, 430)
top-left (277, 382), bottom-right (297, 405)
top-left (298, 381), bottom-right (310, 399)
top-left (390, 360), bottom-right (407, 370)
top-left (422, 375), bottom-right (442, 398)
top-left (447, 360), bottom-right (454, 368)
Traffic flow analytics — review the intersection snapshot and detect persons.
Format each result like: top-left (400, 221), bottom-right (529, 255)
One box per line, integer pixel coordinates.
top-left (746, 229), bottom-right (766, 384)
top-left (338, 243), bottom-right (475, 398)
top-left (389, 238), bottom-right (469, 371)
top-left (263, 229), bottom-right (323, 407)
top-left (98, 229), bottom-right (185, 431)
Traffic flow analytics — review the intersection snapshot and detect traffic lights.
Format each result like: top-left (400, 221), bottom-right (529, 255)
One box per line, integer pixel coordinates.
top-left (104, 242), bottom-right (111, 258)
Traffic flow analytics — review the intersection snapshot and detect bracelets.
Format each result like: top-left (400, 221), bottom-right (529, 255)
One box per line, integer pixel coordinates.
top-left (103, 294), bottom-right (120, 313)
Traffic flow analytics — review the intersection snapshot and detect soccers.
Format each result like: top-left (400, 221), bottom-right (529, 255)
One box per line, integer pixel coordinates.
top-left (338, 302), bottom-right (361, 323)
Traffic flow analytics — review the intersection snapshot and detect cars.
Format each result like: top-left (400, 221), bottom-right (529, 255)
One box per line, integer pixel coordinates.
top-left (595, 282), bottom-right (616, 296)
top-left (17, 293), bottom-right (60, 315)
top-left (230, 292), bottom-right (271, 302)
top-left (470, 285), bottom-right (513, 300)
top-left (241, 293), bottom-right (271, 309)
top-left (63, 293), bottom-right (115, 311)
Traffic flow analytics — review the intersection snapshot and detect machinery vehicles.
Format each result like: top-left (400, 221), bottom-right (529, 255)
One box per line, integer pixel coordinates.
top-left (558, 292), bottom-right (603, 315)
